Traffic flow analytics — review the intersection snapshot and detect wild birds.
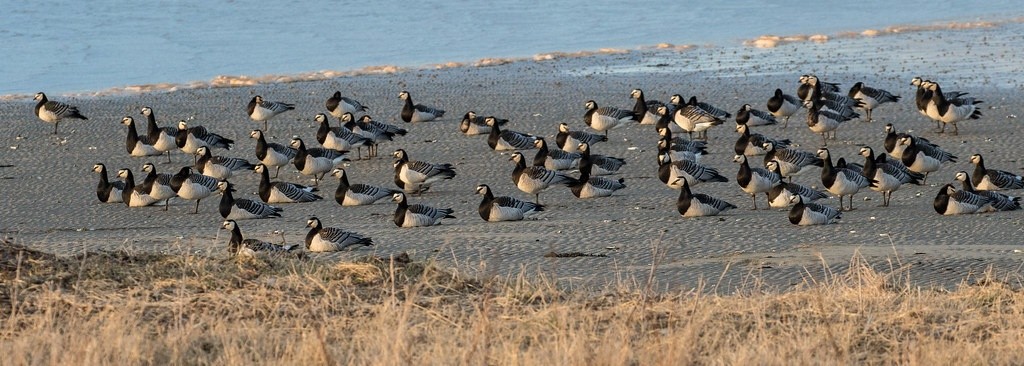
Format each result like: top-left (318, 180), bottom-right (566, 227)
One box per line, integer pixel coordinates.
top-left (460, 111), bottom-right (510, 137)
top-left (248, 93), bottom-right (296, 133)
top-left (785, 194), bottom-right (843, 224)
top-left (396, 90), bottom-right (446, 126)
top-left (392, 192), bottom-right (458, 229)
top-left (669, 174), bottom-right (737, 218)
top-left (734, 155), bottom-right (784, 211)
top-left (475, 183), bottom-right (541, 224)
top-left (32, 91), bottom-right (85, 132)
top-left (208, 177), bottom-right (279, 220)
top-left (816, 147), bottom-right (879, 211)
top-left (223, 220), bottom-right (300, 262)
top-left (506, 150), bottom-right (574, 195)
top-left (330, 164), bottom-right (402, 212)
top-left (93, 105), bottom-right (408, 238)
top-left (737, 77), bottom-right (1024, 219)
top-left (326, 91), bottom-right (369, 121)
top-left (304, 217), bottom-right (371, 258)
top-left (473, 88), bottom-right (732, 200)
top-left (387, 148), bottom-right (459, 197)
